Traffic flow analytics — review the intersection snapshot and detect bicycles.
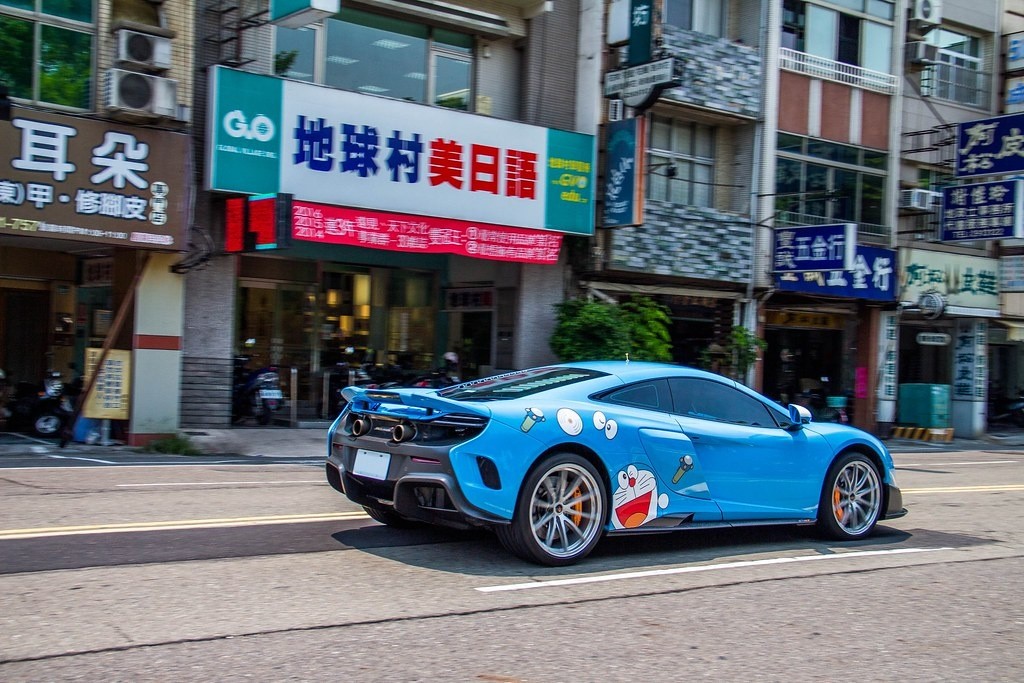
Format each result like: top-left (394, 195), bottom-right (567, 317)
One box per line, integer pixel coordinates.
top-left (793, 392), bottom-right (848, 424)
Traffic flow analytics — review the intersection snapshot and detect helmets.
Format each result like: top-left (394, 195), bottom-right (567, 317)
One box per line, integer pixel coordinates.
top-left (444, 351), bottom-right (458, 363)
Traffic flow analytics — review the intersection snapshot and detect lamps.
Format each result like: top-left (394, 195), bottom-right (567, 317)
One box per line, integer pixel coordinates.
top-left (646, 162), bottom-right (679, 177)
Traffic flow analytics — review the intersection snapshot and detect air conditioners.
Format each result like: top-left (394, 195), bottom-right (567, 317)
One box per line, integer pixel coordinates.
top-left (101, 68), bottom-right (178, 118)
top-left (909, 0), bottom-right (942, 25)
top-left (903, 40), bottom-right (940, 64)
top-left (114, 28), bottom-right (173, 71)
top-left (896, 190), bottom-right (932, 211)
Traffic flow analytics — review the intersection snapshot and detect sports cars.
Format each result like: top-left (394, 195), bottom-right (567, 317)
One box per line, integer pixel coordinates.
top-left (326, 359), bottom-right (911, 566)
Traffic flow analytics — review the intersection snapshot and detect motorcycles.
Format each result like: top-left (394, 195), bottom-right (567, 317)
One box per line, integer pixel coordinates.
top-left (987, 379), bottom-right (1024, 428)
top-left (0, 362), bottom-right (79, 436)
top-left (232, 352), bottom-right (284, 426)
top-left (329, 351), bottom-right (468, 419)
